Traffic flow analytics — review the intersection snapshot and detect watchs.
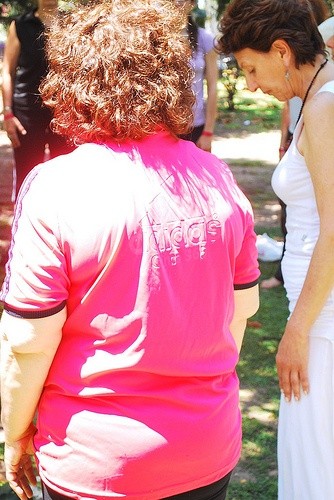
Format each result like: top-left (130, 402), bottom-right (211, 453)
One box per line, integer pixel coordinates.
top-left (4, 106), bottom-right (13, 111)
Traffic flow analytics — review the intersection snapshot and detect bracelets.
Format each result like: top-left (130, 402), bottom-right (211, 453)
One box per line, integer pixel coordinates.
top-left (3, 113), bottom-right (14, 120)
top-left (202, 130), bottom-right (213, 137)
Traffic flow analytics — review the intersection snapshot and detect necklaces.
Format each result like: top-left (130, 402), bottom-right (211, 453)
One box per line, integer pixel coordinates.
top-left (284, 59), bottom-right (328, 153)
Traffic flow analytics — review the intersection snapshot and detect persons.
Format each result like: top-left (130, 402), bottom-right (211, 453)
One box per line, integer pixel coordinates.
top-left (171, 0), bottom-right (218, 153)
top-left (213, 0), bottom-right (334, 500)
top-left (1, 2), bottom-right (75, 225)
top-left (0, 0), bottom-right (261, 500)
top-left (260, 1), bottom-right (334, 287)
